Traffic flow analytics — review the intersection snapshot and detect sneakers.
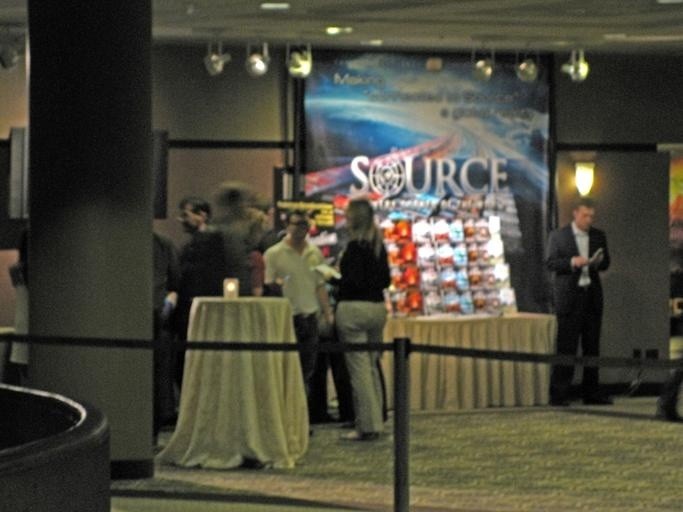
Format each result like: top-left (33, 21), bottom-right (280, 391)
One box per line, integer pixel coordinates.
top-left (339, 431), bottom-right (378, 440)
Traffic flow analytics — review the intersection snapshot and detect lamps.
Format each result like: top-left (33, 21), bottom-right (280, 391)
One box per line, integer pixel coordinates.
top-left (469, 42), bottom-right (590, 84)
top-left (575, 162), bottom-right (595, 197)
top-left (203, 40), bottom-right (313, 78)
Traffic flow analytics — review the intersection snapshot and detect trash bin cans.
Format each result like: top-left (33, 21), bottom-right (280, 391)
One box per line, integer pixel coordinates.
top-left (0, 384), bottom-right (111, 512)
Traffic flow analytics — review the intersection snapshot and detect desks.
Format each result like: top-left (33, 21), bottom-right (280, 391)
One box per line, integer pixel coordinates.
top-left (185, 298), bottom-right (294, 467)
top-left (383, 312), bottom-right (558, 410)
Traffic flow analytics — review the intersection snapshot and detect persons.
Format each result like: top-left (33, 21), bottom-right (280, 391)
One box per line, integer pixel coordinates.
top-left (546, 197), bottom-right (613, 407)
top-left (152, 181), bottom-right (391, 441)
top-left (657, 366), bottom-right (683, 423)
